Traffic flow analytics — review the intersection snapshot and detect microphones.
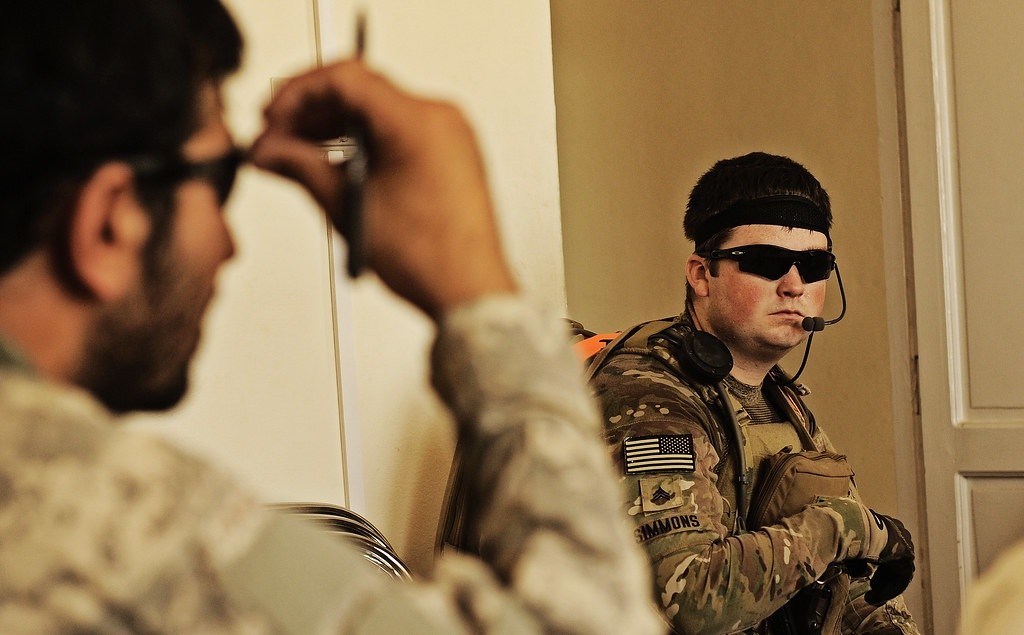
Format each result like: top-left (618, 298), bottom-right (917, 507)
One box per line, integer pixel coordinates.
top-left (801, 264), bottom-right (846, 332)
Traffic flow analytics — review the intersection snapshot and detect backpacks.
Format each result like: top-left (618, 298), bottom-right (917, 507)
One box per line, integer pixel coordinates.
top-left (434, 319), bottom-right (735, 589)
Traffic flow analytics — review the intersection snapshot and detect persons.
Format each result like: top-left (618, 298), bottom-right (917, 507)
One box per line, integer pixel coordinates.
top-left (0, 0), bottom-right (671, 634)
top-left (585, 153), bottom-right (918, 635)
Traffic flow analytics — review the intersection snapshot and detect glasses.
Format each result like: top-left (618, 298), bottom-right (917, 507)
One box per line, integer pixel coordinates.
top-left (132, 143), bottom-right (239, 210)
top-left (693, 244), bottom-right (834, 286)
